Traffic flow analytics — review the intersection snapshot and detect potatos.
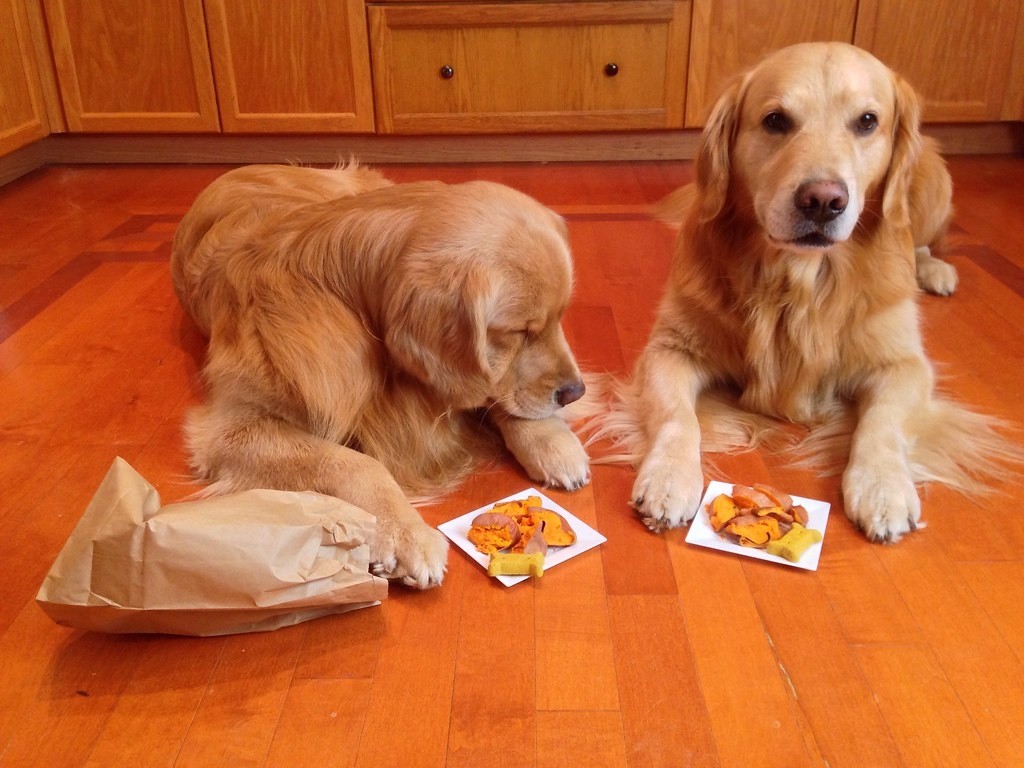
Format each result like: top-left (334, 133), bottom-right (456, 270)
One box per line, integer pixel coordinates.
top-left (467, 494), bottom-right (577, 555)
top-left (708, 483), bottom-right (810, 548)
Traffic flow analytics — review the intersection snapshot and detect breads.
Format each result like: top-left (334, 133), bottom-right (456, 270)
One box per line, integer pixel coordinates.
top-left (487, 551), bottom-right (545, 577)
top-left (766, 522), bottom-right (822, 562)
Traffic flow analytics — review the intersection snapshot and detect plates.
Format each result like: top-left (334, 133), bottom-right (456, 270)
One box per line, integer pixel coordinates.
top-left (436, 487), bottom-right (608, 588)
top-left (684, 480), bottom-right (831, 572)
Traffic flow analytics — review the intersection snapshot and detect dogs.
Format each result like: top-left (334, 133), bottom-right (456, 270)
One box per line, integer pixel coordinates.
top-left (574, 42), bottom-right (1024, 546)
top-left (170, 151), bottom-right (617, 590)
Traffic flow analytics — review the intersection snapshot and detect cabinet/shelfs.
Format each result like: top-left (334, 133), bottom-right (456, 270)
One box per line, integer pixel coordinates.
top-left (0, 0), bottom-right (1024, 187)
